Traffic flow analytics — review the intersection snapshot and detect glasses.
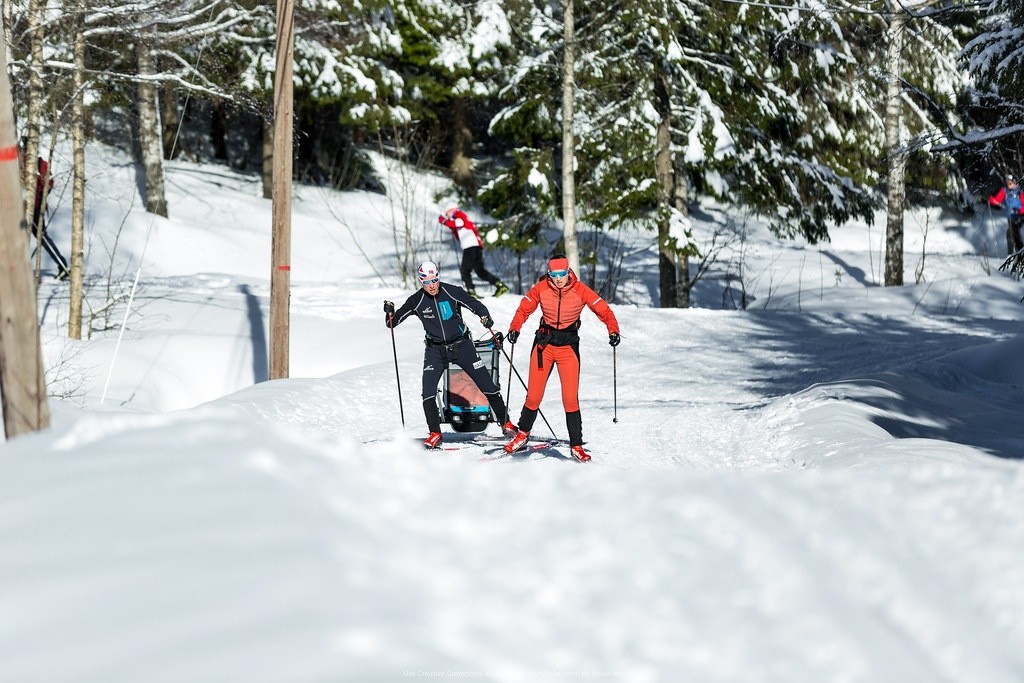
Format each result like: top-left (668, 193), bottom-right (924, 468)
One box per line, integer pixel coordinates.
top-left (548, 267), bottom-right (569, 278)
top-left (418, 275), bottom-right (439, 285)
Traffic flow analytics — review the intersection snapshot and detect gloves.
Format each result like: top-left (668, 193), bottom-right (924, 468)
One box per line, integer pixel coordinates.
top-left (384, 300), bottom-right (394, 314)
top-left (480, 316), bottom-right (494, 328)
top-left (508, 330), bottom-right (520, 343)
top-left (609, 332), bottom-right (620, 346)
top-left (439, 215), bottom-right (445, 223)
top-left (479, 241), bottom-right (483, 248)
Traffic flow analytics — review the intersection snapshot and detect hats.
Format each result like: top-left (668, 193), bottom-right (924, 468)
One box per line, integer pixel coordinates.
top-left (548, 254), bottom-right (568, 271)
top-left (445, 202), bottom-right (458, 214)
top-left (418, 261), bottom-right (439, 280)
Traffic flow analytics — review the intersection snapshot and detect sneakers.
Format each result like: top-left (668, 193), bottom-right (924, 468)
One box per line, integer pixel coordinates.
top-left (468, 290), bottom-right (477, 298)
top-left (503, 431), bottom-right (530, 452)
top-left (502, 422), bottom-right (519, 438)
top-left (492, 282), bottom-right (509, 297)
top-left (423, 432), bottom-right (442, 448)
top-left (571, 445), bottom-right (591, 463)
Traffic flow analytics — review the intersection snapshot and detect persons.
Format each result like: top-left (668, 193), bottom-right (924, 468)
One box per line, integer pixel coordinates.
top-left (384, 261), bottom-right (519, 448)
top-left (18, 135), bottom-right (70, 281)
top-left (990, 174), bottom-right (1024, 255)
top-left (503, 254), bottom-right (620, 463)
top-left (438, 203), bottom-right (509, 299)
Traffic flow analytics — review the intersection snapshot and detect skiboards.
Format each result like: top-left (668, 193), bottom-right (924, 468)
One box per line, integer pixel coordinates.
top-left (478, 438), bottom-right (596, 466)
top-left (416, 435), bottom-right (590, 453)
